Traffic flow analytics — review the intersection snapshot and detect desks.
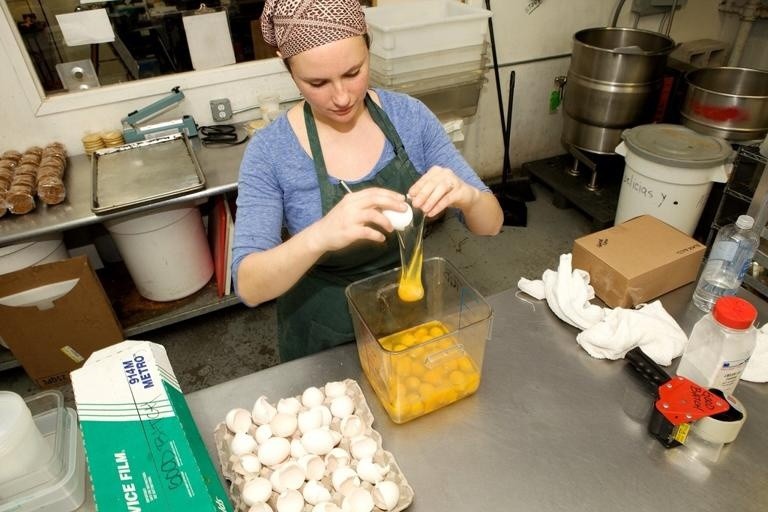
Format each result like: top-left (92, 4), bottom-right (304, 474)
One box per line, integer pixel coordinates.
top-left (69, 262), bottom-right (766, 512)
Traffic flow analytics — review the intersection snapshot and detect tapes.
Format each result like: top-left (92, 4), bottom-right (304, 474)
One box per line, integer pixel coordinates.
top-left (0, 232), bottom-right (70, 276)
top-left (692, 387), bottom-right (747, 444)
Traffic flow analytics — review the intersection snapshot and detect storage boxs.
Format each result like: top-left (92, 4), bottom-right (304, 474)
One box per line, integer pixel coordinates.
top-left (571, 214), bottom-right (707, 309)
top-left (0, 340), bottom-right (235, 512)
top-left (364, 0), bottom-right (494, 125)
top-left (344, 256), bottom-right (493, 426)
top-left (0, 244), bottom-right (125, 390)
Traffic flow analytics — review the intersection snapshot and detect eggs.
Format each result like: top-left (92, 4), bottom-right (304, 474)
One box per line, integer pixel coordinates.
top-left (357, 211), bottom-right (481, 423)
top-left (224, 382), bottom-right (355, 466)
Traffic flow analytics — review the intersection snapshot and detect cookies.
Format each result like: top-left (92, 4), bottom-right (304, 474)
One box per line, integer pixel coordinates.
top-left (83, 130), bottom-right (124, 157)
top-left (0, 143), bottom-right (66, 218)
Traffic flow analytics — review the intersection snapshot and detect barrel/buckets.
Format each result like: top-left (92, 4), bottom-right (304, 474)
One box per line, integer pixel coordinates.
top-left (0, 234), bottom-right (73, 349)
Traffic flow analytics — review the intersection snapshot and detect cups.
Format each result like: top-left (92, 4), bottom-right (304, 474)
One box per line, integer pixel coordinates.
top-left (258, 92), bottom-right (280, 124)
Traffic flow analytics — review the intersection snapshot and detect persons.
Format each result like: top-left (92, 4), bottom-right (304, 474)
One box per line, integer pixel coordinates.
top-left (226, 2), bottom-right (508, 364)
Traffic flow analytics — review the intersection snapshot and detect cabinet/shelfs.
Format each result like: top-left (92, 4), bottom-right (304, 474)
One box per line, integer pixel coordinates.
top-left (0, 118), bottom-right (270, 373)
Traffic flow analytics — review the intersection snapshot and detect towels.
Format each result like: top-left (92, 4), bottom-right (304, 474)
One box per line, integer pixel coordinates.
top-left (516, 254), bottom-right (687, 366)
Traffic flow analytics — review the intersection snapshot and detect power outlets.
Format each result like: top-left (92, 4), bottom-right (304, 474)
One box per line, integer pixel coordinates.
top-left (209, 98), bottom-right (232, 122)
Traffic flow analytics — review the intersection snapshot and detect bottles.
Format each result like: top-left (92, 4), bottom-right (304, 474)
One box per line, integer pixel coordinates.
top-left (693, 213), bottom-right (760, 317)
top-left (675, 295), bottom-right (757, 397)
